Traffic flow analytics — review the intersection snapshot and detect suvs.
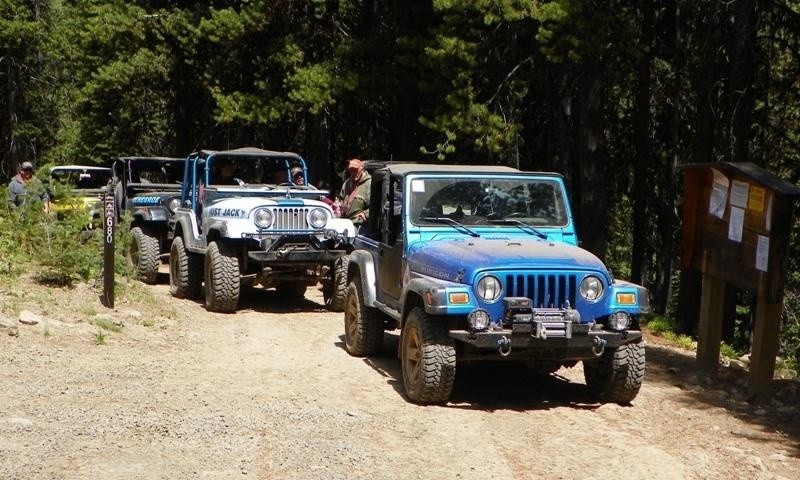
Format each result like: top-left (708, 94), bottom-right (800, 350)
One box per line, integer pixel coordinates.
top-left (109, 156), bottom-right (186, 282)
top-left (336, 168), bottom-right (649, 404)
top-left (168, 149), bottom-right (360, 314)
top-left (50, 166), bottom-right (113, 229)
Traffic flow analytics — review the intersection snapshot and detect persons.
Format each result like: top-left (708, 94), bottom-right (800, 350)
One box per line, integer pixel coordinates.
top-left (212, 157), bottom-right (249, 186)
top-left (292, 166), bottom-right (342, 219)
top-left (337, 158), bottom-right (374, 231)
top-left (6, 159), bottom-right (50, 218)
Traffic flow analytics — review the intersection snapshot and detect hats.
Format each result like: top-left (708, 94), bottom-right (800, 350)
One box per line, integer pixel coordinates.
top-left (22, 162), bottom-right (34, 171)
top-left (349, 159), bottom-right (363, 169)
top-left (291, 167), bottom-right (303, 177)
